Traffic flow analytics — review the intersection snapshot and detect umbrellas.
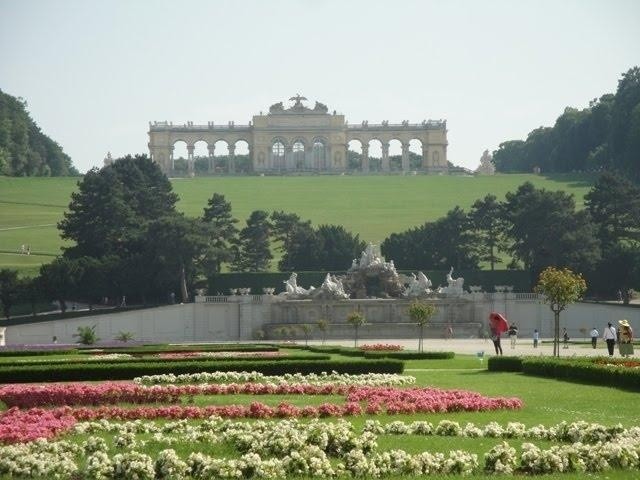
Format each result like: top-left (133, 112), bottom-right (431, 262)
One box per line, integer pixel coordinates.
top-left (489, 312), bottom-right (508, 333)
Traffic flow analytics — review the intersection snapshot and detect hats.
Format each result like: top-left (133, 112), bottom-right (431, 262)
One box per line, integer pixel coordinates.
top-left (619, 319), bottom-right (630, 327)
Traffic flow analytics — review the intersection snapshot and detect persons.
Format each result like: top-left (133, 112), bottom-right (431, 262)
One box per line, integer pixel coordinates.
top-left (617, 320), bottom-right (635, 356)
top-left (489, 317), bottom-right (504, 356)
top-left (590, 327), bottom-right (600, 349)
top-left (603, 321), bottom-right (617, 356)
top-left (563, 327), bottom-right (568, 349)
top-left (509, 321), bottom-right (518, 349)
top-left (448, 324), bottom-right (454, 338)
top-left (283, 242), bottom-right (467, 300)
top-left (533, 329), bottom-right (538, 348)
